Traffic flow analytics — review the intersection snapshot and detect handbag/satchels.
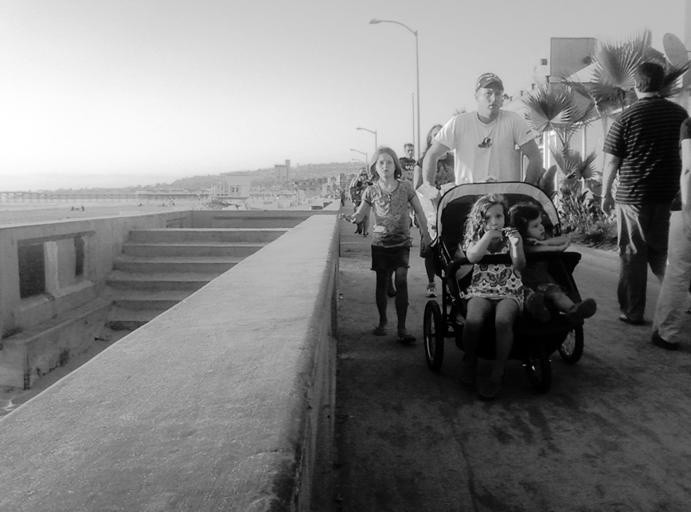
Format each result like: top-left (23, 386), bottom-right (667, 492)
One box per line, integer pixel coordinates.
top-left (382, 234), bottom-right (408, 250)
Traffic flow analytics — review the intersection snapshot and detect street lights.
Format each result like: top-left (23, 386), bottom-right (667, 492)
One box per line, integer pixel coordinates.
top-left (355, 125), bottom-right (379, 157)
top-left (350, 148), bottom-right (369, 173)
top-left (370, 18), bottom-right (422, 170)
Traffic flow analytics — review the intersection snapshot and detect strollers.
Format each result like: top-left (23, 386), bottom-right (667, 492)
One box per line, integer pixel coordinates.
top-left (421, 178), bottom-right (587, 376)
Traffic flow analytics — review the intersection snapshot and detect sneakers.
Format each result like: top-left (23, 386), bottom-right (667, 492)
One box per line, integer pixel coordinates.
top-left (424, 281), bottom-right (437, 297)
top-left (620, 312), bottom-right (642, 325)
top-left (377, 321), bottom-right (388, 335)
top-left (652, 329), bottom-right (679, 349)
top-left (477, 370), bottom-right (504, 398)
top-left (526, 292), bottom-right (550, 324)
top-left (398, 328), bottom-right (416, 341)
top-left (565, 299), bottom-right (596, 326)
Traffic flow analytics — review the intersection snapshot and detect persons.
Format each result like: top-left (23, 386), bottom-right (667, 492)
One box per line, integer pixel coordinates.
top-left (339, 142), bottom-right (430, 299)
top-left (652, 117), bottom-right (691, 353)
top-left (462, 190), bottom-right (529, 400)
top-left (507, 198), bottom-right (598, 331)
top-left (424, 72), bottom-right (545, 188)
top-left (347, 146), bottom-right (435, 346)
top-left (413, 124), bottom-right (455, 298)
top-left (600, 61), bottom-right (690, 327)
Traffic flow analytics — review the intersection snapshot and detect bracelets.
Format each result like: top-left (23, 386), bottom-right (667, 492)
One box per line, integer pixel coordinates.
top-left (599, 195), bottom-right (614, 199)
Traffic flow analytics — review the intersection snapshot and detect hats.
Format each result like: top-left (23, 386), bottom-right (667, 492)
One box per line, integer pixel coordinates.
top-left (475, 72), bottom-right (504, 91)
